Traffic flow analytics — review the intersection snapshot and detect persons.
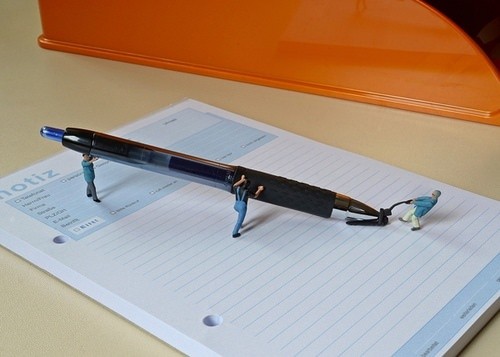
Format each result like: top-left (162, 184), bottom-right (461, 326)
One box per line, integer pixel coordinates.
top-left (399, 190), bottom-right (441, 231)
top-left (81, 152), bottom-right (101, 203)
top-left (231, 175), bottom-right (264, 238)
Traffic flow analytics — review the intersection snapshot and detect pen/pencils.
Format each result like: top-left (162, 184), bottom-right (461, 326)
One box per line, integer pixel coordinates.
top-left (40, 126), bottom-right (379, 216)
